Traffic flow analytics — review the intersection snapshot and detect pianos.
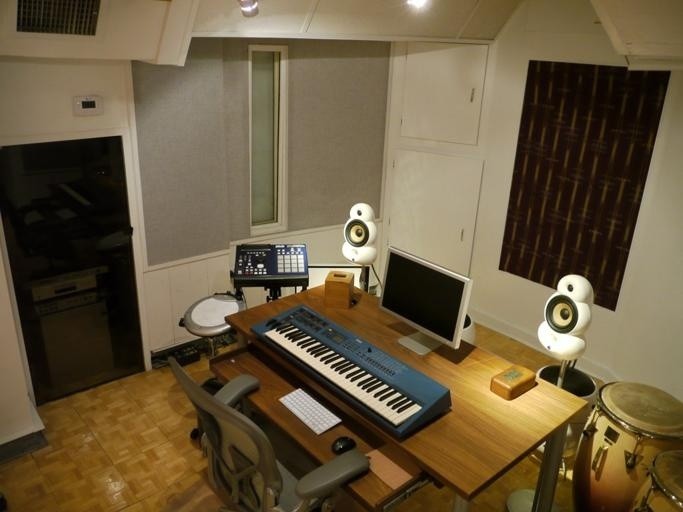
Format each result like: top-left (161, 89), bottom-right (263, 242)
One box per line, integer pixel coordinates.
top-left (249, 303), bottom-right (451, 439)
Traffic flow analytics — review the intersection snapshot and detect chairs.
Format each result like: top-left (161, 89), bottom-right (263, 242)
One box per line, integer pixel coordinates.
top-left (166, 355), bottom-right (372, 511)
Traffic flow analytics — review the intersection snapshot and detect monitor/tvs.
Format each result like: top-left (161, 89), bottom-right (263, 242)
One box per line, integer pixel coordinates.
top-left (379, 246), bottom-right (473, 355)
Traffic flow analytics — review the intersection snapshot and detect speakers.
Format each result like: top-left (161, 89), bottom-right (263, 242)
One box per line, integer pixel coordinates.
top-left (538, 274), bottom-right (594, 361)
top-left (341, 202), bottom-right (377, 265)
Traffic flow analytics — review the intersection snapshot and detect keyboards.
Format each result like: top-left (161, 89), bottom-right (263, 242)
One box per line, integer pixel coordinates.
top-left (278, 387), bottom-right (342, 435)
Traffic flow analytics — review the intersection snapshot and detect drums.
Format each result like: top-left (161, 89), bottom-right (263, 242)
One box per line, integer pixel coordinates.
top-left (572, 380), bottom-right (682, 511)
top-left (184, 294), bottom-right (247, 337)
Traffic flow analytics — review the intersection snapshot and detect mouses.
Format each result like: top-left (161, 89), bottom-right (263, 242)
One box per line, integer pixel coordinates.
top-left (331, 435), bottom-right (356, 455)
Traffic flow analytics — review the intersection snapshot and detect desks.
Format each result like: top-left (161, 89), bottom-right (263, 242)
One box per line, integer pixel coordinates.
top-left (208, 283), bottom-right (590, 511)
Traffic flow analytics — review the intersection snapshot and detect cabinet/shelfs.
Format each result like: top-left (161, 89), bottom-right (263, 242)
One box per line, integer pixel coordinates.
top-left (379, 38), bottom-right (487, 276)
